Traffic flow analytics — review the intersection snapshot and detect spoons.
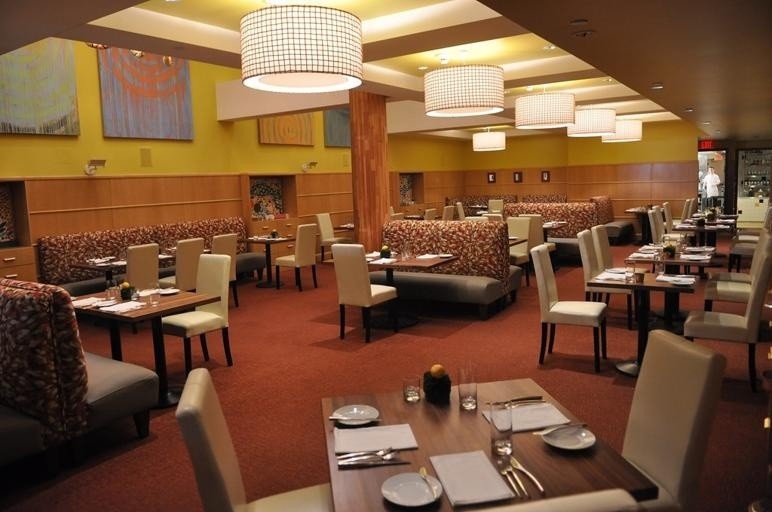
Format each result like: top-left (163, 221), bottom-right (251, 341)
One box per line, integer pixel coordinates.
top-left (486, 396), bottom-right (546, 407)
top-left (532, 423), bottom-right (588, 435)
top-left (419, 464), bottom-right (437, 499)
top-left (336, 446), bottom-right (411, 466)
top-left (497, 456), bottom-right (545, 498)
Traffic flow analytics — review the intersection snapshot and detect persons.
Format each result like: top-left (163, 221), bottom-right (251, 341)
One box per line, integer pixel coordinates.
top-left (703, 167), bottom-right (720, 207)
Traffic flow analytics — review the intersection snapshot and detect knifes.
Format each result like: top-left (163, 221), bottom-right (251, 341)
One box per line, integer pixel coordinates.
top-left (329, 417), bottom-right (384, 422)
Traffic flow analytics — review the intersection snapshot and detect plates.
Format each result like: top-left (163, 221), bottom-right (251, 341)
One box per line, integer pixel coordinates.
top-left (341, 223), bottom-right (354, 228)
top-left (542, 221), bottom-right (567, 228)
top-left (381, 472), bottom-right (442, 507)
top-left (248, 229), bottom-right (293, 241)
top-left (509, 236), bottom-right (518, 240)
top-left (439, 254), bottom-right (453, 257)
top-left (367, 258), bottom-right (374, 262)
top-left (608, 269), bottom-right (625, 273)
top-left (675, 214), bottom-right (736, 229)
top-left (333, 405), bottom-right (379, 425)
top-left (631, 245), bottom-right (715, 260)
top-left (541, 424), bottom-right (596, 450)
top-left (86, 256), bottom-right (116, 267)
top-left (668, 279), bottom-right (696, 285)
top-left (69, 281), bottom-right (180, 313)
top-left (167, 247), bottom-right (176, 254)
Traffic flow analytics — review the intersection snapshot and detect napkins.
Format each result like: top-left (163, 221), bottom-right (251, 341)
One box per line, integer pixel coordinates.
top-left (333, 424), bottom-right (420, 454)
top-left (481, 402), bottom-right (570, 433)
top-left (430, 451), bottom-right (515, 507)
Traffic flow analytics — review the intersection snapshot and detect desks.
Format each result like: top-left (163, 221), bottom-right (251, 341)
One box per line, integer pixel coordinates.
top-left (74, 290), bottom-right (222, 409)
top-left (242, 235), bottom-right (297, 289)
top-left (321, 378), bottom-right (659, 512)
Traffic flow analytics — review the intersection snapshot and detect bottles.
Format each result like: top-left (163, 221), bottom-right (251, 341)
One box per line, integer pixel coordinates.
top-left (423, 373), bottom-right (451, 406)
top-left (746, 157), bottom-right (771, 186)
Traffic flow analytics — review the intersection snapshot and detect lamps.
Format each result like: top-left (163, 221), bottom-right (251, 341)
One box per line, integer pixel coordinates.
top-left (565, 103), bottom-right (617, 139)
top-left (301, 160), bottom-right (319, 173)
top-left (513, 83), bottom-right (575, 131)
top-left (472, 129), bottom-right (507, 153)
top-left (600, 117), bottom-right (644, 144)
top-left (239, 2), bottom-right (363, 96)
top-left (84, 158), bottom-right (106, 176)
top-left (423, 46), bottom-right (506, 118)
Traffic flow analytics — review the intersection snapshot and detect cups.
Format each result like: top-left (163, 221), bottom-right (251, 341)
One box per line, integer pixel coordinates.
top-left (489, 401), bottom-right (513, 455)
top-left (458, 368), bottom-right (477, 410)
top-left (624, 260), bottom-right (635, 282)
top-left (403, 376), bottom-right (421, 402)
top-left (400, 251), bottom-right (411, 261)
top-left (656, 262), bottom-right (665, 277)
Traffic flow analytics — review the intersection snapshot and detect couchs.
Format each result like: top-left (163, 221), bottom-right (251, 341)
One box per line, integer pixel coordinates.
top-left (2, 278), bottom-right (160, 464)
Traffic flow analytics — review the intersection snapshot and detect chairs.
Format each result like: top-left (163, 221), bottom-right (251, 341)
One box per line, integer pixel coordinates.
top-left (620, 329), bottom-right (727, 511)
top-left (161, 254), bottom-right (233, 377)
top-left (685, 248), bottom-right (772, 390)
top-left (34, 217), bottom-right (267, 308)
top-left (486, 489), bottom-right (640, 511)
top-left (592, 196), bottom-right (771, 335)
top-left (176, 368), bottom-right (334, 512)
top-left (335, 193), bottom-right (635, 318)
top-left (275, 222), bottom-right (317, 291)
top-left (530, 244), bottom-right (607, 373)
top-left (318, 214), bottom-right (349, 263)
top-left (331, 243), bottom-right (397, 343)
